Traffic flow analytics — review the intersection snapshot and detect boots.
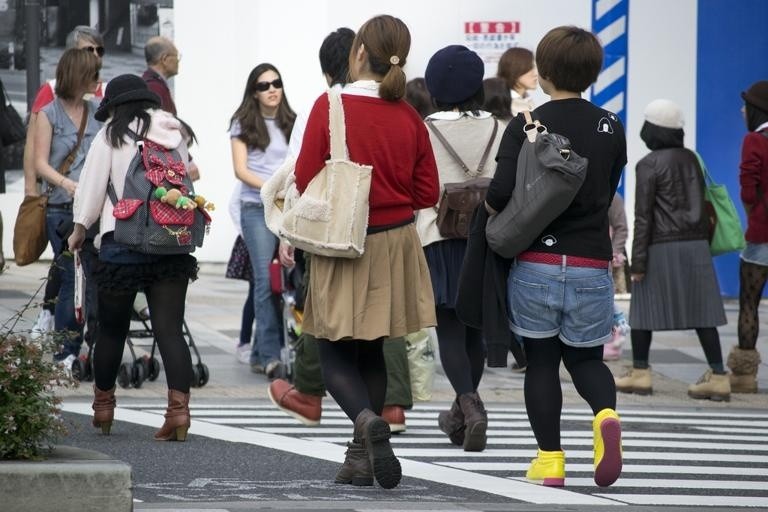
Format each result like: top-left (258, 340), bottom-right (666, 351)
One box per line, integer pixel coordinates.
top-left (155, 389), bottom-right (205, 441)
top-left (334, 408), bottom-right (401, 488)
top-left (602, 311), bottom-right (631, 360)
top-left (439, 392), bottom-right (487, 451)
top-left (93, 384), bottom-right (116, 435)
top-left (727, 346), bottom-right (760, 393)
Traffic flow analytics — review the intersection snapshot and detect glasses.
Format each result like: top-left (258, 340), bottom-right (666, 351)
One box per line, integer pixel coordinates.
top-left (255, 79), bottom-right (283, 92)
top-left (94, 71), bottom-right (99, 81)
top-left (81, 46), bottom-right (104, 57)
top-left (167, 53), bottom-right (182, 61)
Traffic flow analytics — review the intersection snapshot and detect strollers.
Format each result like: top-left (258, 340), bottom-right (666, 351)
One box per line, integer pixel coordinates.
top-left (72, 241), bottom-right (209, 388)
top-left (275, 251), bottom-right (433, 402)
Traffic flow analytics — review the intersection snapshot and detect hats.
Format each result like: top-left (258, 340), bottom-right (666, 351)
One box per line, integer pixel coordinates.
top-left (94, 74), bottom-right (161, 122)
top-left (645, 99), bottom-right (685, 130)
top-left (741, 81), bottom-right (768, 112)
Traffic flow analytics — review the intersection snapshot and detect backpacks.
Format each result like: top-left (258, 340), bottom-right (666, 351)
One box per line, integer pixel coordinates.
top-left (425, 115), bottom-right (498, 239)
top-left (107, 122), bottom-right (205, 255)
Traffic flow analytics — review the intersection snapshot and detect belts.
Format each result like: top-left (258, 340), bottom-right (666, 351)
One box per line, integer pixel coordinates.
top-left (517, 251), bottom-right (608, 268)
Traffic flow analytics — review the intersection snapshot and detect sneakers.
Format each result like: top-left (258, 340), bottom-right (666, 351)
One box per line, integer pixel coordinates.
top-left (381, 406), bottom-right (406, 433)
top-left (613, 365), bottom-right (652, 395)
top-left (251, 360), bottom-right (287, 381)
top-left (688, 370), bottom-right (730, 401)
top-left (268, 379), bottom-right (321, 426)
top-left (592, 408), bottom-right (622, 487)
top-left (526, 446), bottom-right (565, 486)
top-left (233, 343), bottom-right (252, 364)
top-left (31, 309), bottom-right (55, 352)
top-left (50, 354), bottom-right (77, 378)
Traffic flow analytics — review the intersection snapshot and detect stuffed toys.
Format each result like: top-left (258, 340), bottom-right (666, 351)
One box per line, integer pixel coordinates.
top-left (154, 184), bottom-right (200, 212)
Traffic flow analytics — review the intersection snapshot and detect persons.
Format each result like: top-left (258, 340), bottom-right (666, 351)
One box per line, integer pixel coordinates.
top-left (141, 34), bottom-right (180, 115)
top-left (293, 12), bottom-right (444, 489)
top-left (22, 26), bottom-right (111, 344)
top-left (613, 100), bottom-right (733, 404)
top-left (65, 72), bottom-right (200, 444)
top-left (727, 80), bottom-right (768, 393)
top-left (227, 26), bottom-right (630, 451)
top-left (481, 25), bottom-right (628, 489)
top-left (32, 46), bottom-right (115, 385)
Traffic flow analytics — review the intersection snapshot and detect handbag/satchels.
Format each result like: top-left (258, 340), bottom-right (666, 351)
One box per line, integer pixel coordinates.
top-left (703, 184), bottom-right (746, 254)
top-left (485, 111), bottom-right (589, 259)
top-left (279, 160), bottom-right (373, 260)
top-left (1, 105), bottom-right (26, 147)
top-left (14, 193), bottom-right (48, 266)
